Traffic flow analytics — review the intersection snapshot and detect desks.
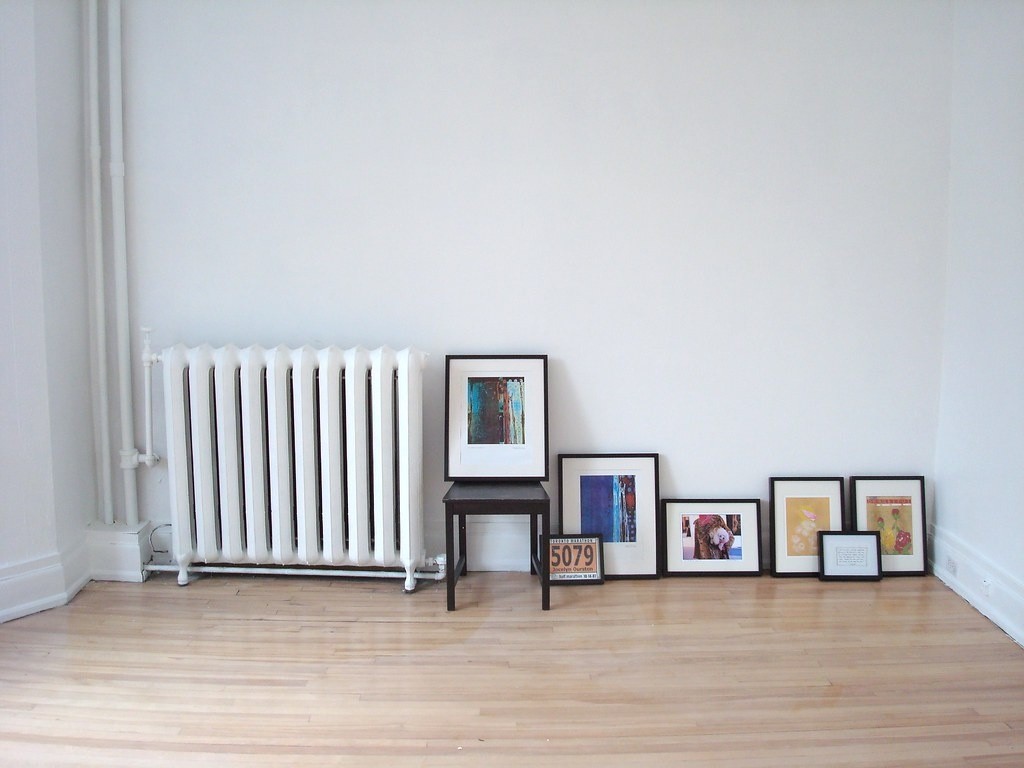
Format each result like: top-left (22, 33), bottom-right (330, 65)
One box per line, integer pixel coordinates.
top-left (442, 480), bottom-right (551, 611)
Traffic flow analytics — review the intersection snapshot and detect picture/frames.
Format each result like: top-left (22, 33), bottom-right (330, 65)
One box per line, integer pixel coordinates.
top-left (444, 355), bottom-right (549, 483)
top-left (817, 529), bottom-right (885, 582)
top-left (849, 475), bottom-right (926, 576)
top-left (538, 533), bottom-right (606, 587)
top-left (557, 452), bottom-right (661, 581)
top-left (768, 475), bottom-right (847, 580)
top-left (661, 497), bottom-right (763, 577)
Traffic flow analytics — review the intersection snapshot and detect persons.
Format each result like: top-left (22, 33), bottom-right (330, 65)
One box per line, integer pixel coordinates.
top-left (693, 514), bottom-right (735, 560)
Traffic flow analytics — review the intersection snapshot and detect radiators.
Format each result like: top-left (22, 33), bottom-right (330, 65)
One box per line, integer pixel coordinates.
top-left (162, 344), bottom-right (428, 592)
